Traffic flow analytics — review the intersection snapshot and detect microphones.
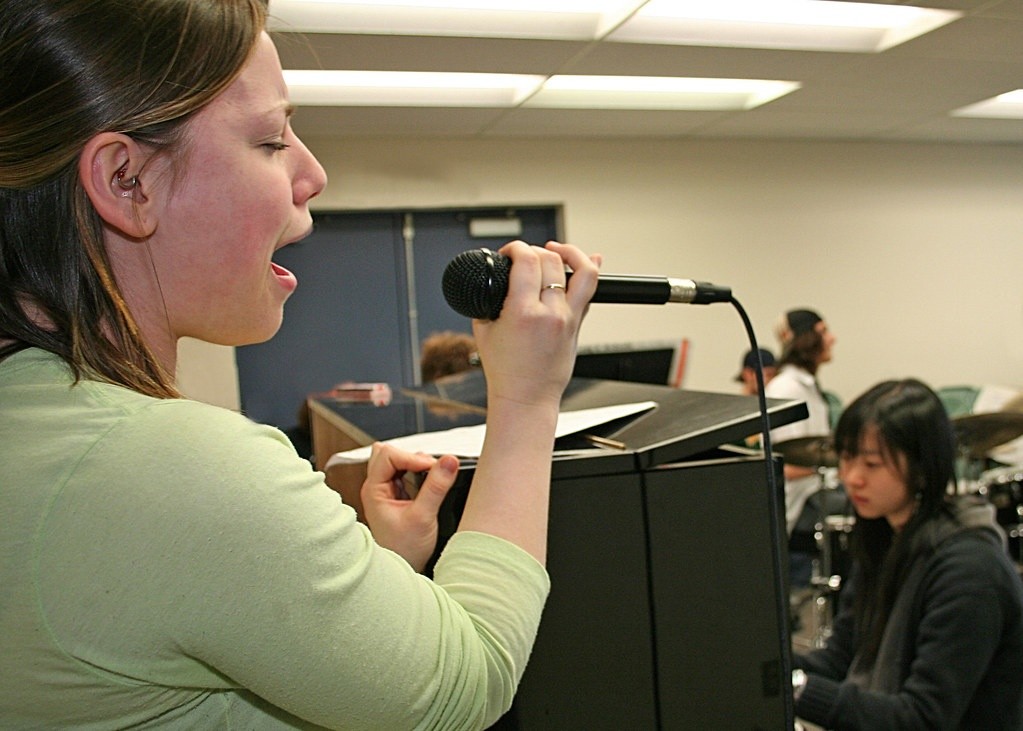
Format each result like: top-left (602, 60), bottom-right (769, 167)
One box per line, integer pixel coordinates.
top-left (441, 247), bottom-right (732, 322)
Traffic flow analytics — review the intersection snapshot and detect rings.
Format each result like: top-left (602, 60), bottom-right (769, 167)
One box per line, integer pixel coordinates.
top-left (541, 284), bottom-right (565, 291)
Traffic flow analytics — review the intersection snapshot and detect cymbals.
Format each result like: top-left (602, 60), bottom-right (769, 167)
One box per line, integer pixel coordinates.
top-left (950, 412), bottom-right (1023, 447)
top-left (775, 435), bottom-right (840, 468)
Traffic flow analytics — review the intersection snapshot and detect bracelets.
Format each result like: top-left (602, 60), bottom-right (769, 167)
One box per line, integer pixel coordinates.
top-left (792, 669), bottom-right (806, 702)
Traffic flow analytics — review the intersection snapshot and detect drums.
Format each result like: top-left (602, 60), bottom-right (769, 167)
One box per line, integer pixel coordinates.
top-left (970, 462), bottom-right (1023, 564)
top-left (812, 516), bottom-right (857, 590)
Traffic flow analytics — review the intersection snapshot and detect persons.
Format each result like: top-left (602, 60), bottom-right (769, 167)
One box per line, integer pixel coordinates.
top-left (0, 1), bottom-right (603, 731)
top-left (792, 378), bottom-right (1023, 731)
top-left (736, 309), bottom-right (835, 543)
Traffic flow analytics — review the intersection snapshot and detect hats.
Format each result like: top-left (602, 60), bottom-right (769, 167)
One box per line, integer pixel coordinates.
top-left (735, 349), bottom-right (776, 381)
top-left (774, 307), bottom-right (826, 344)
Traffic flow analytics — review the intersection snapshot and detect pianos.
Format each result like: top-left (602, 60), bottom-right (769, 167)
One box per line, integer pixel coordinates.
top-left (304, 362), bottom-right (816, 731)
